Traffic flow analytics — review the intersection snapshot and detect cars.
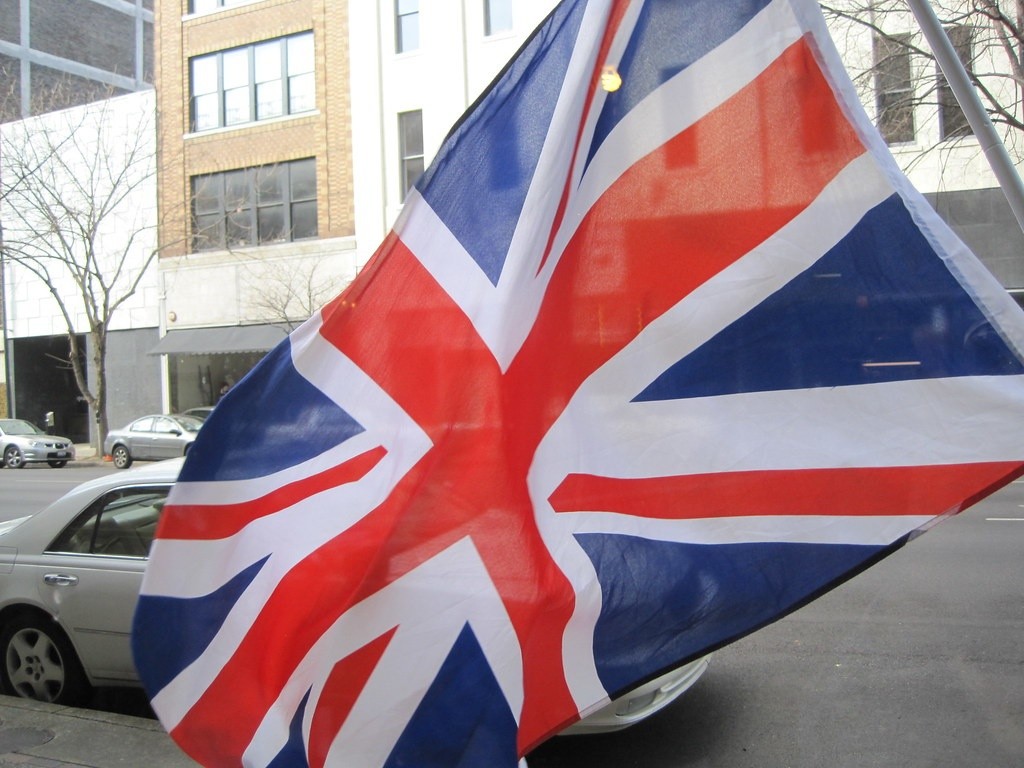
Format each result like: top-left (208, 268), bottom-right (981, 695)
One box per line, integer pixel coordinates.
top-left (265, 403), bottom-right (365, 457)
top-left (183, 407), bottom-right (216, 422)
top-left (104, 413), bottom-right (207, 469)
top-left (0, 455), bottom-right (710, 737)
top-left (0, 418), bottom-right (77, 469)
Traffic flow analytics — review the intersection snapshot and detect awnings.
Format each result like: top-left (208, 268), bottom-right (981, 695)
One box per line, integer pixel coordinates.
top-left (144, 321), bottom-right (308, 355)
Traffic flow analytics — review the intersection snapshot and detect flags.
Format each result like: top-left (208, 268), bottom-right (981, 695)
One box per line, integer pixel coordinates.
top-left (130, 0), bottom-right (1022, 768)
